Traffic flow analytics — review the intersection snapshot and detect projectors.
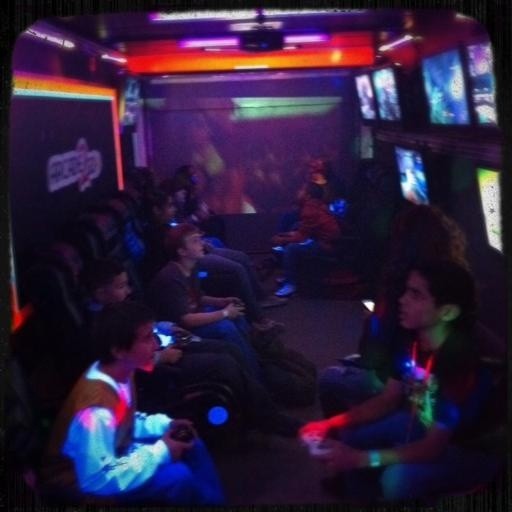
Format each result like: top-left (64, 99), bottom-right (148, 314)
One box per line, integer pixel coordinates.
top-left (237, 28), bottom-right (285, 52)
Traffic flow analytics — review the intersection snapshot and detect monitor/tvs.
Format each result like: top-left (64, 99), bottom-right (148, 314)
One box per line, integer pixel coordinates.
top-left (389, 143), bottom-right (432, 209)
top-left (472, 159), bottom-right (503, 258)
top-left (349, 61), bottom-right (410, 132)
top-left (418, 37), bottom-right (499, 131)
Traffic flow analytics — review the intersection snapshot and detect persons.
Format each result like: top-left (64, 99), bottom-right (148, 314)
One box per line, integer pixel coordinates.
top-left (1, 159), bottom-right (511, 510)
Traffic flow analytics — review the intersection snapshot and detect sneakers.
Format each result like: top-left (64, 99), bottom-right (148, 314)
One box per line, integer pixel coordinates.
top-left (256, 294), bottom-right (290, 308)
